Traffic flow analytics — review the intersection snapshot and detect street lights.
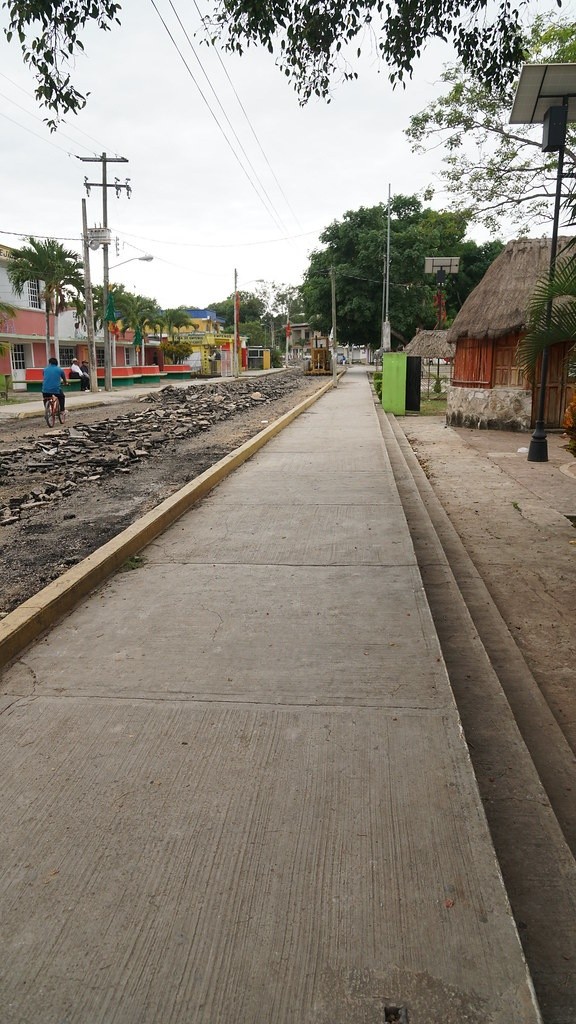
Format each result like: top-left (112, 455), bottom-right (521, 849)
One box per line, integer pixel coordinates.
top-left (84, 239), bottom-right (99, 392)
top-left (286, 313), bottom-right (305, 368)
top-left (234, 280), bottom-right (264, 378)
top-left (103, 256), bottom-right (153, 391)
top-left (260, 324), bottom-right (275, 346)
top-left (526, 97), bottom-right (570, 462)
top-left (436, 270), bottom-right (445, 376)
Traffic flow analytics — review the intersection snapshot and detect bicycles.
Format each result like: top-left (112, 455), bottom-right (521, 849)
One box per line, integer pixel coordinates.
top-left (45, 384), bottom-right (70, 428)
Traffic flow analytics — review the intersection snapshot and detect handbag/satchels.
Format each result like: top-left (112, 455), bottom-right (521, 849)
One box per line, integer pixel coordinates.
top-left (68, 371), bottom-right (80, 379)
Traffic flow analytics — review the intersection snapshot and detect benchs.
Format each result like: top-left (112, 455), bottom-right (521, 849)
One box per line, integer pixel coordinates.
top-left (141, 372), bottom-right (168, 386)
top-left (96, 375), bottom-right (141, 387)
top-left (12, 379), bottom-right (82, 391)
top-left (164, 370), bottom-right (194, 379)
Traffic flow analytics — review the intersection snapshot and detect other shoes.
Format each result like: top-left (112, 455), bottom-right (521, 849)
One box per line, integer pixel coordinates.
top-left (81, 388), bottom-right (85, 391)
top-left (60, 410), bottom-right (68, 416)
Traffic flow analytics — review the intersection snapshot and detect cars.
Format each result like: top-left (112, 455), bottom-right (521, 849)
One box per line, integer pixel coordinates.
top-left (424, 358), bottom-right (450, 365)
top-left (336, 352), bottom-right (349, 363)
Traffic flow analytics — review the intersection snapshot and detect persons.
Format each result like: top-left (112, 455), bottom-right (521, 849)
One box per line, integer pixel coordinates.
top-left (71, 358), bottom-right (90, 390)
top-left (342, 356), bottom-right (346, 365)
top-left (42, 357), bottom-right (69, 415)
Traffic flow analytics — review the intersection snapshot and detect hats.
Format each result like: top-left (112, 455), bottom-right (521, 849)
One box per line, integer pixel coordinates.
top-left (72, 358), bottom-right (78, 363)
top-left (81, 360), bottom-right (89, 364)
